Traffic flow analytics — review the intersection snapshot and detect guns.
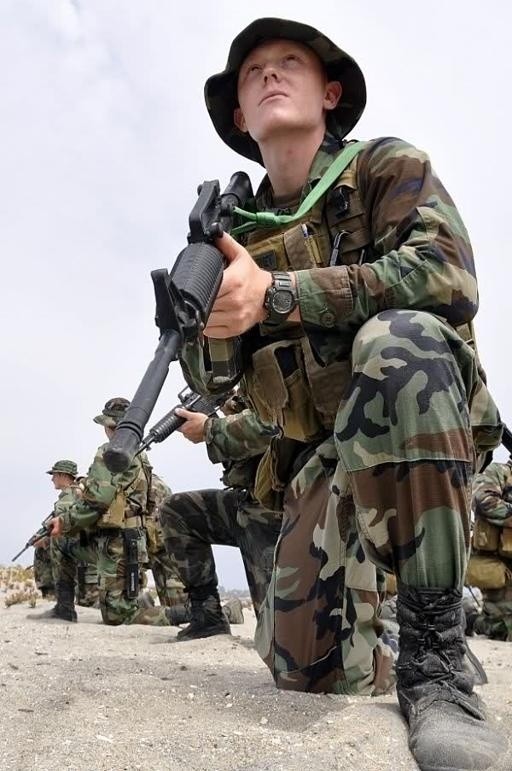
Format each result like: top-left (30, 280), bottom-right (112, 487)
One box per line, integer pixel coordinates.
top-left (104, 171), bottom-right (254, 471)
top-left (135, 383), bottom-right (237, 457)
top-left (12, 512), bottom-right (53, 562)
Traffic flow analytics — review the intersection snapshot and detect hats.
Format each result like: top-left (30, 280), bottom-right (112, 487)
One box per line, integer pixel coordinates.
top-left (93, 397), bottom-right (131, 427)
top-left (203, 17), bottom-right (367, 169)
top-left (46, 460), bottom-right (79, 480)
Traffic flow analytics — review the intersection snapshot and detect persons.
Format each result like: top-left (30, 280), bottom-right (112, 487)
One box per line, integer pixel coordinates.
top-left (461, 458), bottom-right (512, 640)
top-left (27, 379), bottom-right (281, 642)
top-left (203, 17), bottom-right (511, 771)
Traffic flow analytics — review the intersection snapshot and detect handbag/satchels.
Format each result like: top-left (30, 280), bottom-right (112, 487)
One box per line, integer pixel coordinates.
top-left (97, 495), bottom-right (124, 529)
top-left (464, 556), bottom-right (506, 590)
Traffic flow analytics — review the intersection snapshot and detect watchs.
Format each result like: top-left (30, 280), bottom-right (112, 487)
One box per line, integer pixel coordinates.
top-left (264, 271), bottom-right (297, 328)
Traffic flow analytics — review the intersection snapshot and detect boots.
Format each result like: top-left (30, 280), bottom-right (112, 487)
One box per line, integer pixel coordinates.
top-left (26, 581), bottom-right (77, 623)
top-left (396, 589), bottom-right (509, 771)
top-left (171, 584), bottom-right (244, 641)
